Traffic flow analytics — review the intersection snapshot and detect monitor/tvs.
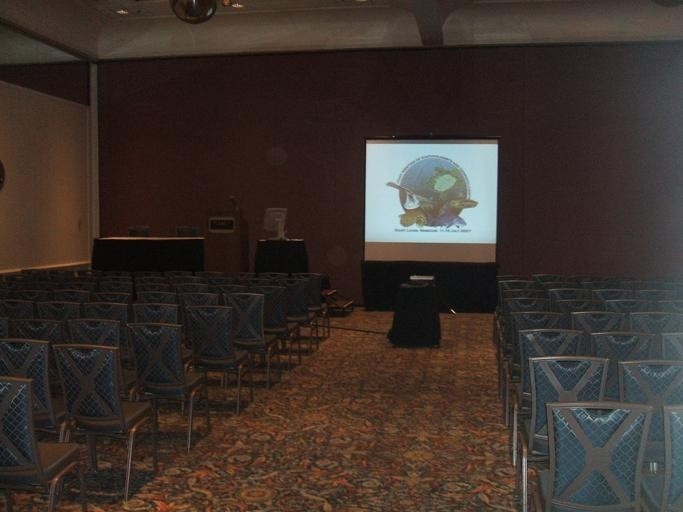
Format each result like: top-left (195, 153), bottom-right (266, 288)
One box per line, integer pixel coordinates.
top-left (262, 208), bottom-right (288, 241)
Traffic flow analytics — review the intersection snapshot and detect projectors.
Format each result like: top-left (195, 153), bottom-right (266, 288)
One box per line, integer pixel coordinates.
top-left (409, 275), bottom-right (434, 284)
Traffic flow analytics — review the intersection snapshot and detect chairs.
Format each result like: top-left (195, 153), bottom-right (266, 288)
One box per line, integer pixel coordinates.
top-left (1, 266), bottom-right (210, 512)
top-left (127, 224), bottom-right (153, 237)
top-left (490, 271), bottom-right (680, 512)
top-left (178, 267), bottom-right (333, 416)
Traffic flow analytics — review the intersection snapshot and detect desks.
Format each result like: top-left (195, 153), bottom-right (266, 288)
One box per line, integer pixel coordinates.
top-left (90, 238), bottom-right (205, 276)
